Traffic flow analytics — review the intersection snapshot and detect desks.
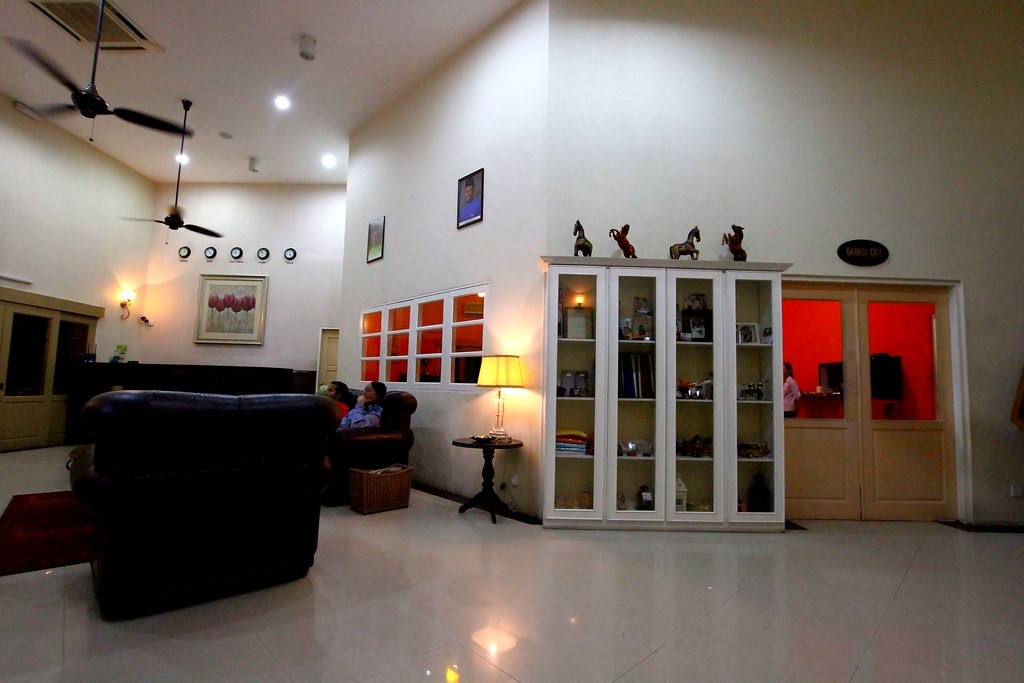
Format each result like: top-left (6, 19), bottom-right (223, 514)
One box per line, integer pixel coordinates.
top-left (452, 439), bottom-right (519, 523)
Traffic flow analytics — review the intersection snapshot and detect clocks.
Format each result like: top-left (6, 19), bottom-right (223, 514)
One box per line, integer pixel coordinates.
top-left (178, 246), bottom-right (297, 260)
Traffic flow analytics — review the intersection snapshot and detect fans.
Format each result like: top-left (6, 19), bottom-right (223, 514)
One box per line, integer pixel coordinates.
top-left (5, 0), bottom-right (195, 140)
top-left (120, 99), bottom-right (223, 240)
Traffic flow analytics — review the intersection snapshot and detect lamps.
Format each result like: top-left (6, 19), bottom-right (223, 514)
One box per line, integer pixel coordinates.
top-left (13, 100), bottom-right (41, 121)
top-left (120, 293), bottom-right (135, 320)
top-left (477, 355), bottom-right (525, 439)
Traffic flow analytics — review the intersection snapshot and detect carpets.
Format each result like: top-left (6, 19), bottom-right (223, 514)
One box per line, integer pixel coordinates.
top-left (0, 491), bottom-right (88, 576)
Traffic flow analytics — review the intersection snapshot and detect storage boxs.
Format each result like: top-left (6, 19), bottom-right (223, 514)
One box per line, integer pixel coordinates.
top-left (349, 465), bottom-right (416, 515)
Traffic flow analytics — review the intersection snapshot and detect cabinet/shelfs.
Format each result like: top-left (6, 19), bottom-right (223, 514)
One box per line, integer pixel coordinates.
top-left (540, 256), bottom-right (796, 533)
top-left (0, 287), bottom-right (105, 452)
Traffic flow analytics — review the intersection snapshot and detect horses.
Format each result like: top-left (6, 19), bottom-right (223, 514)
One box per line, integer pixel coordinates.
top-left (721, 223), bottom-right (747, 262)
top-left (609, 224), bottom-right (639, 258)
top-left (669, 225), bottom-right (702, 261)
top-left (573, 219), bottom-right (594, 258)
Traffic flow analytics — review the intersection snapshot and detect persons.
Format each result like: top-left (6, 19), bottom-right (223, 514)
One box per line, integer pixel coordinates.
top-left (324, 380), bottom-right (349, 482)
top-left (783, 362), bottom-right (802, 418)
top-left (319, 381), bottom-right (387, 506)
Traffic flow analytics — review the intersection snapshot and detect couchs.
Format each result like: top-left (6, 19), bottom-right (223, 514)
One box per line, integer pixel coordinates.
top-left (70, 390), bottom-right (333, 623)
top-left (321, 388), bottom-right (418, 521)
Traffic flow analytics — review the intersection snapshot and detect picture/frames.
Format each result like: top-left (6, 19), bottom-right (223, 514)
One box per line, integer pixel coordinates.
top-left (455, 169), bottom-right (486, 229)
top-left (193, 273), bottom-right (269, 346)
top-left (367, 217), bottom-right (385, 263)
